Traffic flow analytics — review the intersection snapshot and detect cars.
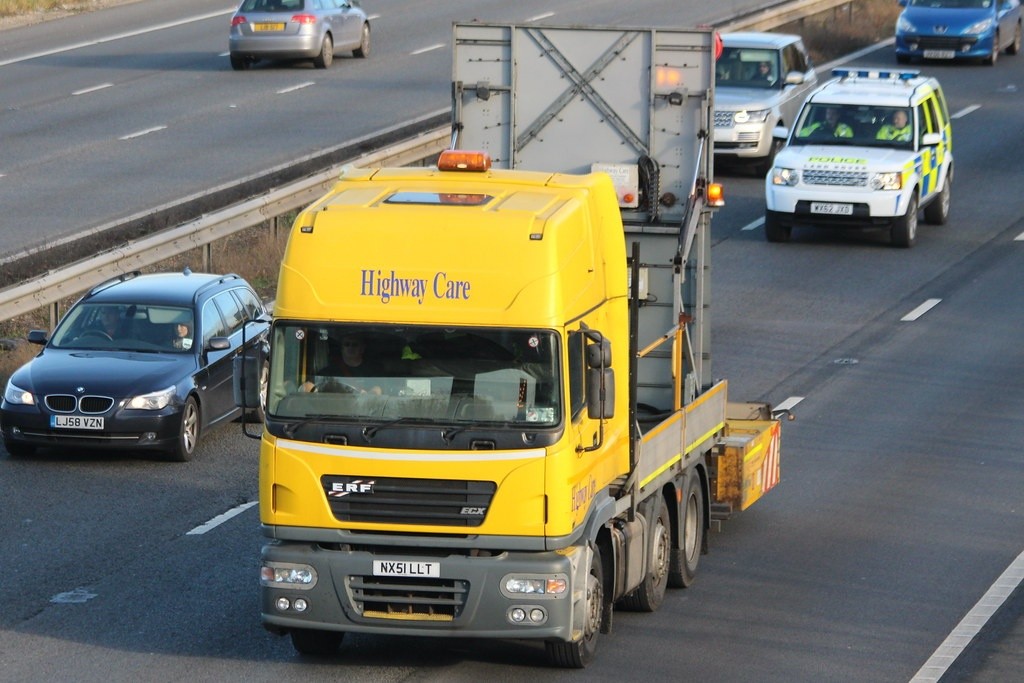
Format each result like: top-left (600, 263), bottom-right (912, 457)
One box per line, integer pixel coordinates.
top-left (4, 269), bottom-right (281, 466)
top-left (232, 0), bottom-right (372, 67)
top-left (764, 66), bottom-right (959, 250)
top-left (893, 0), bottom-right (1023, 69)
top-left (701, 30), bottom-right (820, 174)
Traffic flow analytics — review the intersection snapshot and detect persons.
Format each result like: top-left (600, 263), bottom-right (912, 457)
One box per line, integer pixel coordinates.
top-left (297, 334), bottom-right (383, 395)
top-left (799, 107), bottom-right (853, 138)
top-left (717, 63), bottom-right (731, 80)
top-left (751, 61), bottom-right (772, 81)
top-left (170, 321), bottom-right (192, 350)
top-left (876, 110), bottom-right (911, 142)
top-left (91, 307), bottom-right (127, 340)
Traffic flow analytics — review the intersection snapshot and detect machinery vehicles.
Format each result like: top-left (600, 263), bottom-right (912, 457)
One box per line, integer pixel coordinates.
top-left (231, 17), bottom-right (794, 667)
